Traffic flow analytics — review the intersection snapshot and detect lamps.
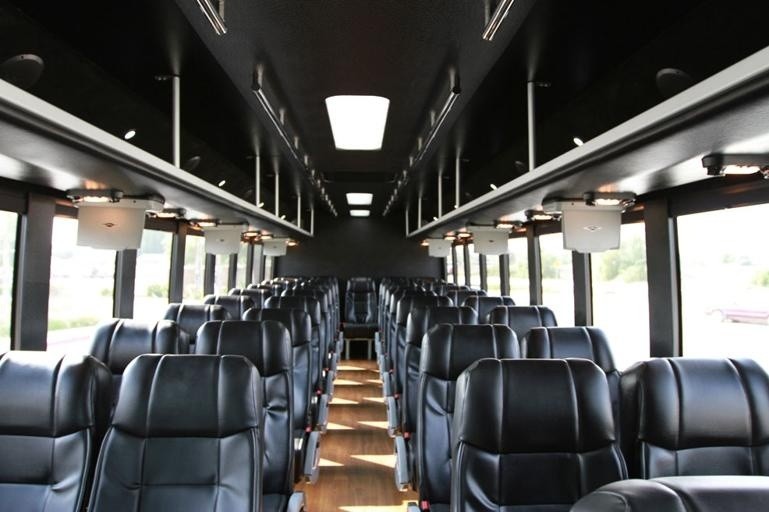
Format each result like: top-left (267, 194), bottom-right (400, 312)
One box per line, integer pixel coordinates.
top-left (73, 129), bottom-right (305, 247)
top-left (324, 93), bottom-right (391, 219)
